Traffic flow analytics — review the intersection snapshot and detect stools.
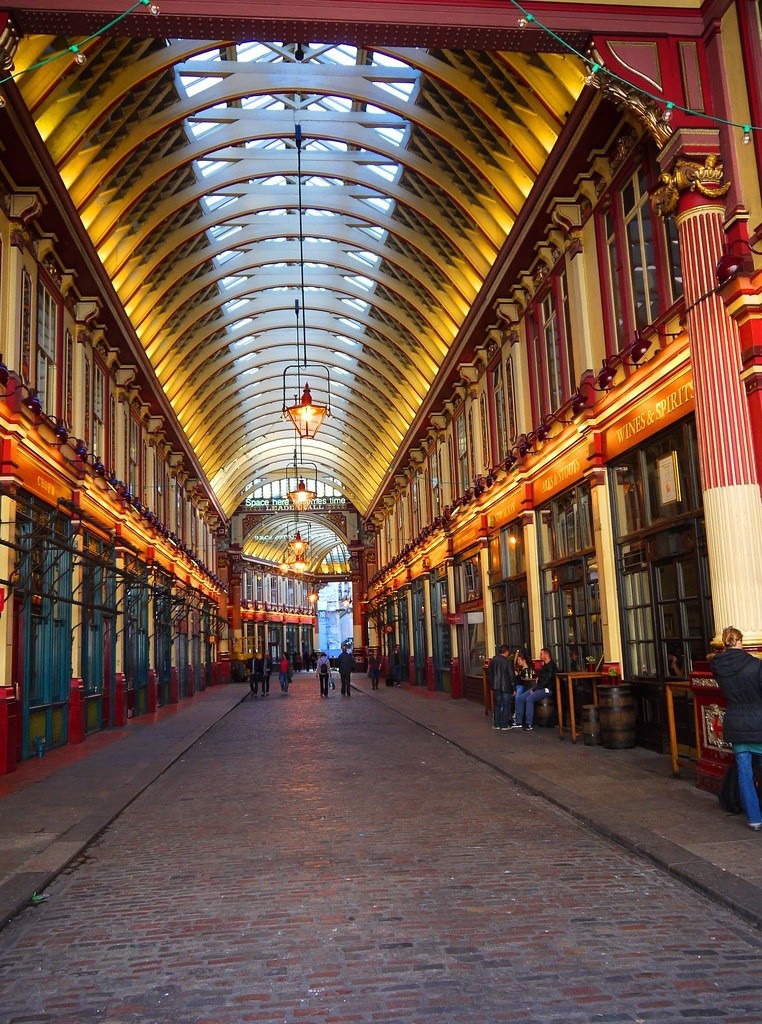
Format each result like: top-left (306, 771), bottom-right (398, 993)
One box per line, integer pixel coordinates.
top-left (535, 697), bottom-right (556, 728)
top-left (583, 705), bottom-right (601, 746)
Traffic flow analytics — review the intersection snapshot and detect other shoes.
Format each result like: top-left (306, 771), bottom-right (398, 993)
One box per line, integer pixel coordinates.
top-left (261, 692), bottom-right (269, 696)
top-left (372, 688), bottom-right (379, 690)
top-left (394, 682), bottom-right (401, 687)
top-left (251, 692), bottom-right (258, 696)
top-left (341, 690), bottom-right (351, 696)
top-left (281, 692), bottom-right (287, 695)
top-left (512, 724), bottom-right (534, 730)
top-left (748, 823), bottom-right (761, 830)
top-left (492, 726), bottom-right (512, 730)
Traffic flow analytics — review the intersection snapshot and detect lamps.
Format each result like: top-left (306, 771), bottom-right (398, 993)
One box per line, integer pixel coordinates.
top-left (279, 395), bottom-right (319, 604)
top-left (0, 353), bottom-right (9, 388)
top-left (286, 300), bottom-right (317, 513)
top-left (367, 414), bottom-right (572, 587)
top-left (571, 382), bottom-right (611, 414)
top-left (50, 437), bottom-right (88, 463)
top-left (630, 324), bottom-right (680, 363)
top-left (68, 454), bottom-right (226, 590)
top-left (715, 239), bottom-right (762, 284)
top-left (0, 384), bottom-right (43, 416)
top-left (35, 415), bottom-right (67, 442)
top-left (598, 355), bottom-right (647, 389)
top-left (282, 125), bottom-right (331, 437)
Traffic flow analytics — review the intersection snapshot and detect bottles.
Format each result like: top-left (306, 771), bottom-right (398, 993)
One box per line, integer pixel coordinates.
top-left (525, 669), bottom-right (528, 678)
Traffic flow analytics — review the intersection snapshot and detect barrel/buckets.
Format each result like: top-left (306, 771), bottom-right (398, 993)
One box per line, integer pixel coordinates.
top-left (535, 699), bottom-right (555, 727)
top-left (596, 683), bottom-right (637, 749)
top-left (581, 705), bottom-right (603, 745)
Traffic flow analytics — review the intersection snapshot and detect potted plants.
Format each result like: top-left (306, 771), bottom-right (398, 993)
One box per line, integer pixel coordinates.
top-left (608, 667), bottom-right (618, 685)
top-left (588, 658), bottom-right (595, 672)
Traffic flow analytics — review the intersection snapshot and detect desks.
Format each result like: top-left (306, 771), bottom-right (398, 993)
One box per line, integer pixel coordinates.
top-left (665, 681), bottom-right (700, 775)
top-left (554, 672), bottom-right (602, 744)
top-left (483, 666), bottom-right (489, 716)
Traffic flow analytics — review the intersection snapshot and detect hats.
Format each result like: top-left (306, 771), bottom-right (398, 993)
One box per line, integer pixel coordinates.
top-left (342, 646), bottom-right (346, 651)
top-left (320, 652), bottom-right (327, 656)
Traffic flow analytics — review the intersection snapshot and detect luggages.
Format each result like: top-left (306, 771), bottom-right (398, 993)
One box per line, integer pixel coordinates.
top-left (386, 668), bottom-right (393, 686)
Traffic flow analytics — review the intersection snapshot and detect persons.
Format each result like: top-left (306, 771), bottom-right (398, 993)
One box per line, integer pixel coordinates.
top-left (247, 644), bottom-right (405, 695)
top-left (711, 627), bottom-right (762, 832)
top-left (488, 644), bottom-right (557, 731)
top-left (669, 652), bottom-right (683, 677)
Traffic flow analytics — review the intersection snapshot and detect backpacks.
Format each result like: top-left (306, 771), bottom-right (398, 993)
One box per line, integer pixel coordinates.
top-left (720, 759), bottom-right (742, 814)
top-left (320, 659), bottom-right (327, 673)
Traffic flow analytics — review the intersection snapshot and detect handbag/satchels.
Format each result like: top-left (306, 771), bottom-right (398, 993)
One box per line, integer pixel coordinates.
top-left (328, 674), bottom-right (335, 690)
